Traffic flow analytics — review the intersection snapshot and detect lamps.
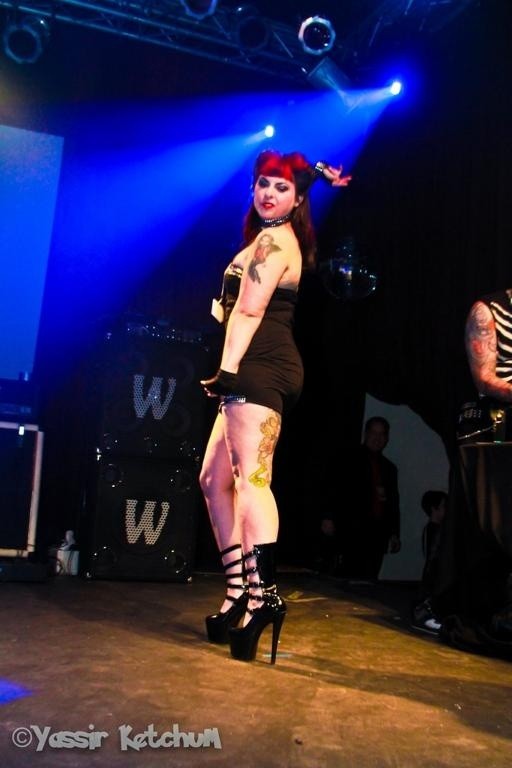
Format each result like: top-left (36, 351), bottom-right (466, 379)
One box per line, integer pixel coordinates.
top-left (305, 57), bottom-right (370, 118)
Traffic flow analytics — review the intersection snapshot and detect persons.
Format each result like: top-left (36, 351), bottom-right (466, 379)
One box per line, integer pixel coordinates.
top-left (200, 148), bottom-right (354, 664)
top-left (418, 486), bottom-right (449, 581)
top-left (320, 414), bottom-right (404, 594)
top-left (426, 271), bottom-right (512, 662)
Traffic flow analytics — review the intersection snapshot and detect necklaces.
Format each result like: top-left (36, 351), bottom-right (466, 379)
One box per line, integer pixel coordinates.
top-left (258, 215), bottom-right (292, 228)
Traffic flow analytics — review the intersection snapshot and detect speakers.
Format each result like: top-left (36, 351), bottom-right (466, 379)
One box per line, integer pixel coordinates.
top-left (79, 331), bottom-right (210, 582)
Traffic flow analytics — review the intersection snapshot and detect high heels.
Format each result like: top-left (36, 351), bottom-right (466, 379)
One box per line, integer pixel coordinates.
top-left (205, 545), bottom-right (246, 643)
top-left (229, 542), bottom-right (286, 663)
